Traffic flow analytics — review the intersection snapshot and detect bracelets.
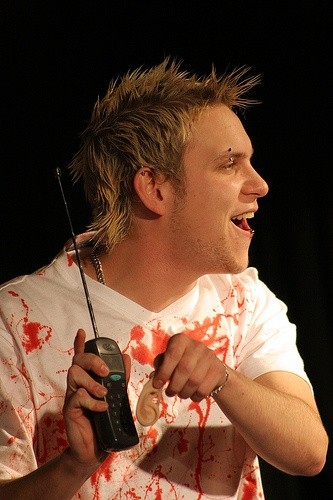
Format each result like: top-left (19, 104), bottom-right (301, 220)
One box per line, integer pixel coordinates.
top-left (208, 361), bottom-right (230, 401)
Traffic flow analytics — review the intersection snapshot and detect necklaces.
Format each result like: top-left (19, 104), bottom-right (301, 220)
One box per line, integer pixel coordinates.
top-left (86, 240), bottom-right (106, 285)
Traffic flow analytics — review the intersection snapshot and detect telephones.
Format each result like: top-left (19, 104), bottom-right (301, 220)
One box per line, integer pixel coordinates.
top-left (55, 165), bottom-right (140, 453)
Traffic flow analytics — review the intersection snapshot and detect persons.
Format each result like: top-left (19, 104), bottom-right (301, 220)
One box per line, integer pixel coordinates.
top-left (0, 59), bottom-right (330, 500)
